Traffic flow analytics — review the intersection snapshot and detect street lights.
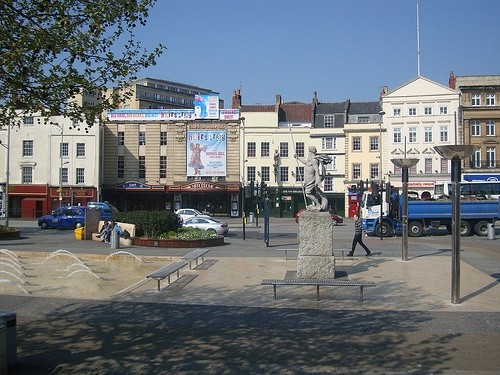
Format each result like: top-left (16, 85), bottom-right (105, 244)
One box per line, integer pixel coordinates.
top-left (52, 122), bottom-right (62, 209)
top-left (433, 110), bottom-right (480, 304)
top-left (242, 159), bottom-right (249, 218)
top-left (0, 139), bottom-right (9, 226)
top-left (390, 136), bottom-right (420, 262)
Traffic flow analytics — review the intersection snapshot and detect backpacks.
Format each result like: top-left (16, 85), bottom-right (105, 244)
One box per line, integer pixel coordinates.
top-left (121, 229), bottom-right (130, 238)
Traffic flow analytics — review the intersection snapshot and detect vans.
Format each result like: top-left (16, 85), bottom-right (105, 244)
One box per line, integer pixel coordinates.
top-left (38, 201), bottom-right (118, 230)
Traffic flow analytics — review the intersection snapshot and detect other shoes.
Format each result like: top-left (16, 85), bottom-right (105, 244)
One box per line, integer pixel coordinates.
top-left (96, 236), bottom-right (100, 238)
top-left (347, 250), bottom-right (353, 256)
top-left (365, 252), bottom-right (371, 256)
top-left (105, 240), bottom-right (111, 244)
top-left (101, 240), bottom-right (105, 242)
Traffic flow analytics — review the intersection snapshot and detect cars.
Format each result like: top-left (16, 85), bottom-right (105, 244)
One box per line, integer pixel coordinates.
top-left (174, 209), bottom-right (211, 224)
top-left (295, 209), bottom-right (343, 226)
top-left (181, 217), bottom-right (229, 235)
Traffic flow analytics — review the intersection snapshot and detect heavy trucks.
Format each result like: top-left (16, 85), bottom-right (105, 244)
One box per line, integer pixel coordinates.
top-left (359, 191), bottom-right (500, 237)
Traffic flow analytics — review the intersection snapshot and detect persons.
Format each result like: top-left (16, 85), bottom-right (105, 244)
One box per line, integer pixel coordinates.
top-left (346, 214), bottom-right (370, 256)
top-left (95, 221), bottom-right (117, 245)
top-left (273, 149), bottom-right (279, 164)
top-left (294, 147), bottom-right (333, 210)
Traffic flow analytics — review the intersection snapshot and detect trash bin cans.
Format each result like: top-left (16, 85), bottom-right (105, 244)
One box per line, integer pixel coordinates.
top-left (249, 212), bottom-right (254, 223)
top-left (110, 230), bottom-right (120, 249)
top-left (0, 310), bottom-right (17, 375)
